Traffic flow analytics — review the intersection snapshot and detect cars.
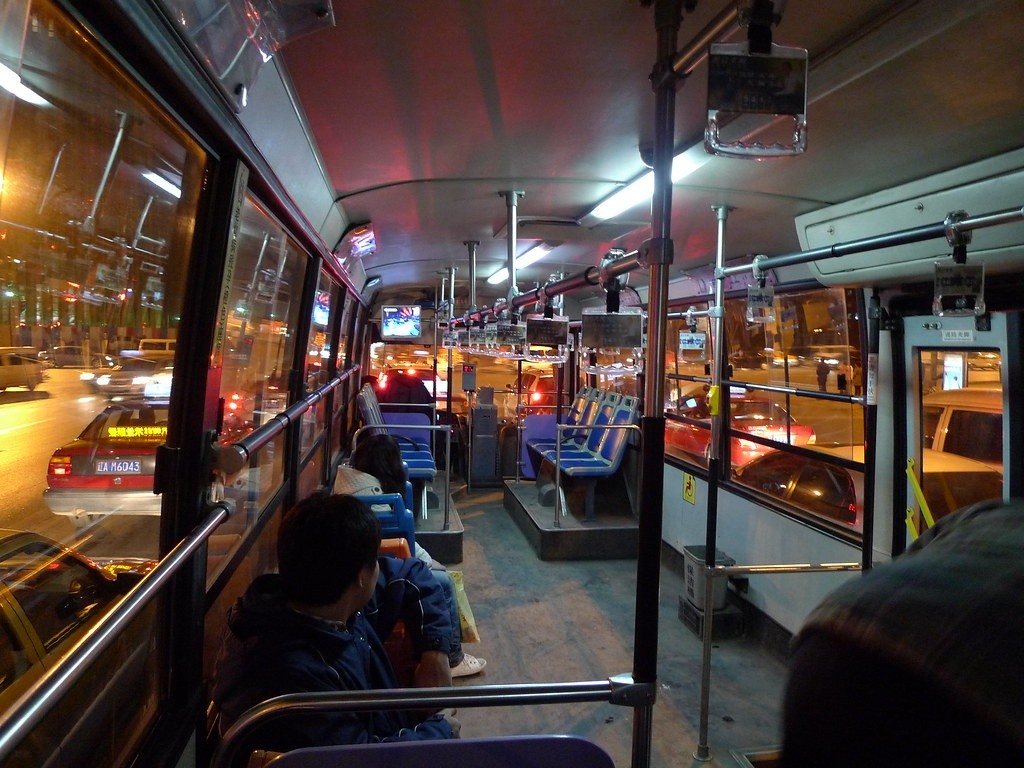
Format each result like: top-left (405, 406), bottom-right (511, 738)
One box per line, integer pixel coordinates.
top-left (730, 441), bottom-right (1003, 536)
top-left (78, 337), bottom-right (177, 403)
top-left (42, 394), bottom-right (254, 528)
top-left (0, 526), bottom-right (158, 768)
top-left (38, 345), bottom-right (119, 369)
top-left (770, 351), bottom-right (800, 369)
top-left (968, 352), bottom-right (1001, 370)
top-left (728, 351), bottom-right (773, 370)
top-left (663, 394), bottom-right (817, 469)
top-left (376, 366), bottom-right (455, 402)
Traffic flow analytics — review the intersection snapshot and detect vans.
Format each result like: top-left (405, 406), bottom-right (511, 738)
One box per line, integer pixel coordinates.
top-left (922, 386), bottom-right (1004, 475)
top-left (0, 345), bottom-right (44, 392)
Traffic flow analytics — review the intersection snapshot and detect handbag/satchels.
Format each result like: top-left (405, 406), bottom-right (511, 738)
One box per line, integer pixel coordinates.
top-left (447, 570), bottom-right (481, 645)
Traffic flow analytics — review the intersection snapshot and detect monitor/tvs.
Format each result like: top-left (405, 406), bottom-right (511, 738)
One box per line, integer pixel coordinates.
top-left (313, 290), bottom-right (331, 328)
top-left (381, 305), bottom-right (422, 339)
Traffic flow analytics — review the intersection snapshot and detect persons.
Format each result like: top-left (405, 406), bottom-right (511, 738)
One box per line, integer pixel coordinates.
top-left (852, 361), bottom-right (862, 396)
top-left (817, 357), bottom-right (830, 393)
top-left (217, 491), bottom-right (459, 752)
top-left (329, 433), bottom-right (488, 679)
top-left (776, 498), bottom-right (1024, 768)
top-left (837, 361), bottom-right (848, 395)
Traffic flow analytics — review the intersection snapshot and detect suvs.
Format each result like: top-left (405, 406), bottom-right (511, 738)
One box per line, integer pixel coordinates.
top-left (503, 366), bottom-right (587, 417)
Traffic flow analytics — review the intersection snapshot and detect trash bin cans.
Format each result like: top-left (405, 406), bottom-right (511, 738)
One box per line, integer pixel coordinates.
top-left (683, 544), bottom-right (734, 609)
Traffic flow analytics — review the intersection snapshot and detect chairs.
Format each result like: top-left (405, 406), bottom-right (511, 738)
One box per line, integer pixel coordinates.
top-left (356, 383), bottom-right (437, 520)
top-left (526, 385), bottom-right (639, 523)
top-left (352, 493), bottom-right (418, 685)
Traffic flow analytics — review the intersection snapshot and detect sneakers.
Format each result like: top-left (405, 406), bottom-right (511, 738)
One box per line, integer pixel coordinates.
top-left (450, 654), bottom-right (487, 679)
